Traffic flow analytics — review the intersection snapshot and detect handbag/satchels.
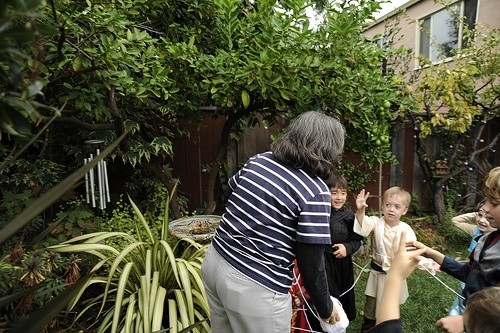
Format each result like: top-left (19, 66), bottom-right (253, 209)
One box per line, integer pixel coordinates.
top-left (313, 295), bottom-right (349, 333)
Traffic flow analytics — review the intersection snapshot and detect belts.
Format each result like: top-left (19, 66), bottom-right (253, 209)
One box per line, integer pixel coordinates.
top-left (371, 261), bottom-right (385, 272)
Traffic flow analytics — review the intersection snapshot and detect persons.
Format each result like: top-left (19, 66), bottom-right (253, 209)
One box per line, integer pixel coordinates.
top-left (199, 111), bottom-right (346, 333)
top-left (405, 167), bottom-right (500, 333)
top-left (364, 231), bottom-right (429, 333)
top-left (448, 196), bottom-right (498, 317)
top-left (323, 174), bottom-right (362, 323)
top-left (353, 187), bottom-right (442, 333)
top-left (461, 286), bottom-right (500, 333)
